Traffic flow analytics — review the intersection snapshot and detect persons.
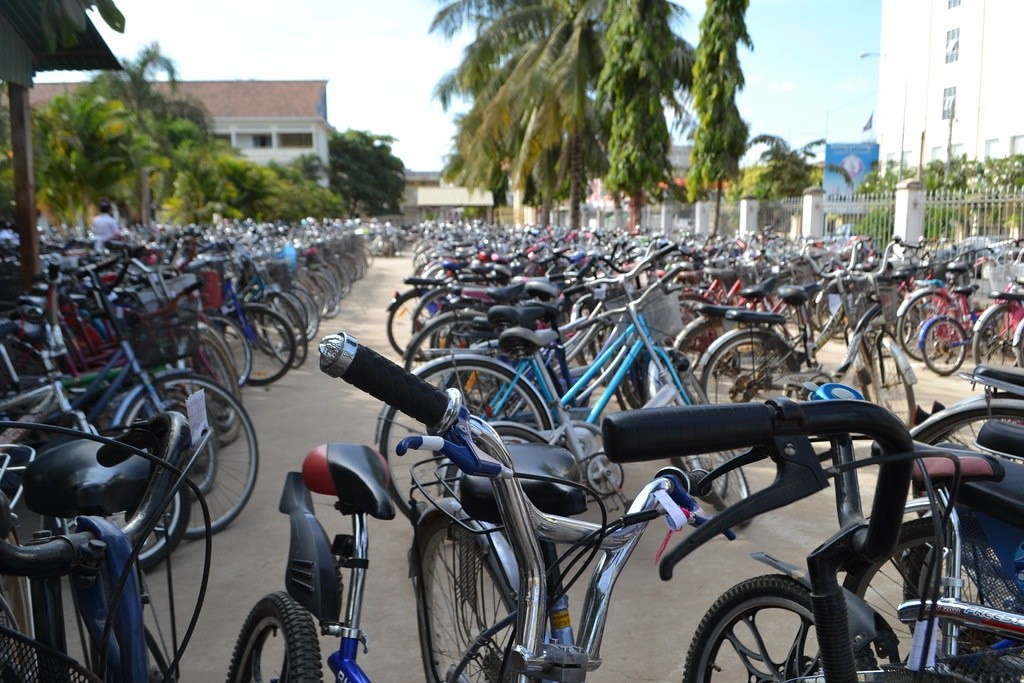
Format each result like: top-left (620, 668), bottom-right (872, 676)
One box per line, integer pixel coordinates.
top-left (0, 221), bottom-right (13, 242)
top-left (92, 201), bottom-right (120, 236)
top-left (36, 208), bottom-right (48, 232)
top-left (293, 215), bottom-right (392, 227)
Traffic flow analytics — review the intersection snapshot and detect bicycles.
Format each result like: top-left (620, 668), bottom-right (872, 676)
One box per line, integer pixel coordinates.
top-left (0, 216), bottom-right (1024, 682)
top-left (215, 330), bottom-right (737, 683)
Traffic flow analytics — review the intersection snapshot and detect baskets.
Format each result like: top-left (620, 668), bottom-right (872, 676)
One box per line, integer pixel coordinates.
top-left (604, 283), bottom-right (684, 345)
top-left (880, 646), bottom-right (1024, 683)
top-left (954, 504), bottom-right (1024, 615)
top-left (125, 296), bottom-right (198, 369)
top-left (723, 266), bottom-right (751, 293)
top-left (988, 263), bottom-right (1024, 291)
top-left (841, 279), bottom-right (897, 330)
top-left (0, 626), bottom-right (103, 683)
